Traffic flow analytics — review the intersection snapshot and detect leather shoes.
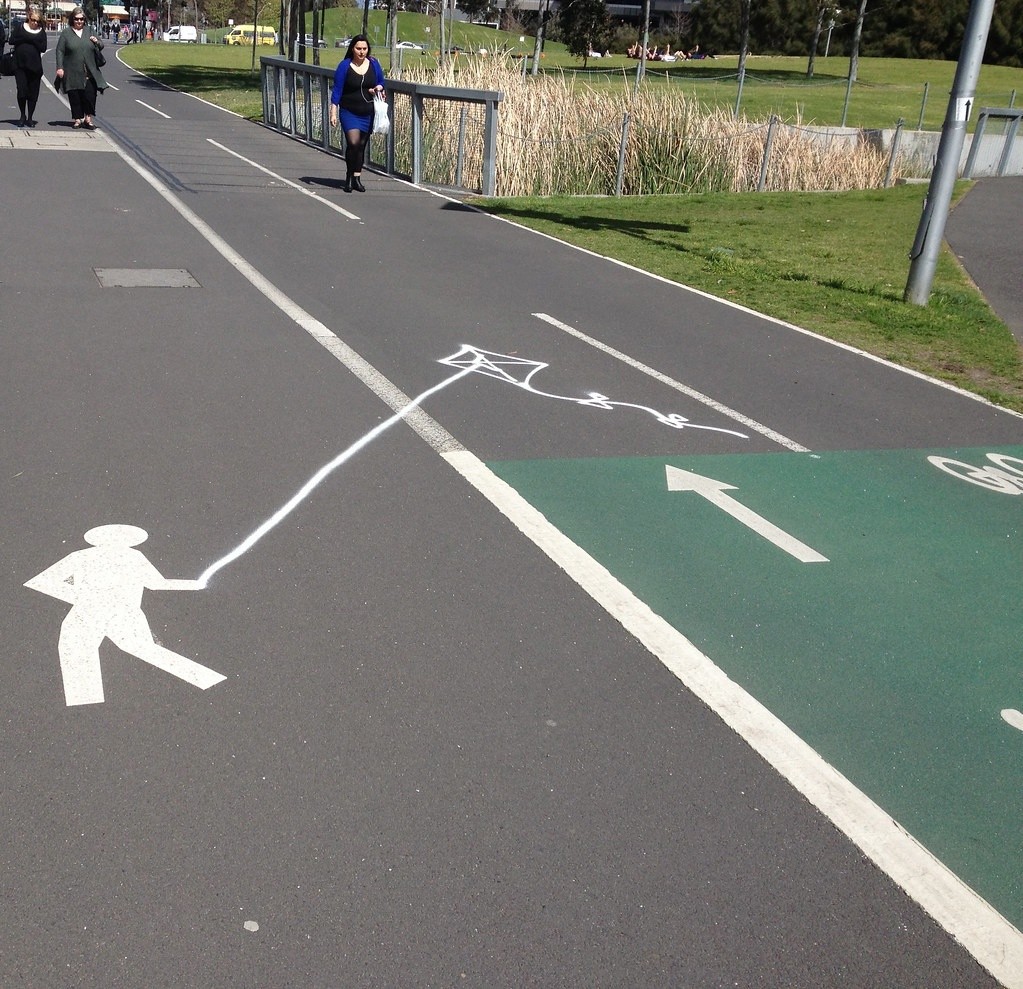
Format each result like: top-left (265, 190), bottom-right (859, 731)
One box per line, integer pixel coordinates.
top-left (353, 176), bottom-right (365, 192)
top-left (344, 177), bottom-right (353, 192)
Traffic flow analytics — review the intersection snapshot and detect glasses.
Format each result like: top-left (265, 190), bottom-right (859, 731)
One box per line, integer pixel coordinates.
top-left (29, 15), bottom-right (41, 23)
top-left (73, 16), bottom-right (85, 21)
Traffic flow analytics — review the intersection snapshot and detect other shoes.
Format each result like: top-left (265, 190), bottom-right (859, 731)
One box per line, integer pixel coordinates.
top-left (26, 120), bottom-right (35, 127)
top-left (73, 122), bottom-right (82, 129)
top-left (84, 120), bottom-right (96, 130)
top-left (18, 115), bottom-right (27, 127)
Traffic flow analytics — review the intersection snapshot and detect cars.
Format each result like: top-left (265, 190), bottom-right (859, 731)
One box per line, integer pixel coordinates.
top-left (297, 33), bottom-right (327, 49)
top-left (335, 38), bottom-right (352, 47)
top-left (395, 41), bottom-right (423, 50)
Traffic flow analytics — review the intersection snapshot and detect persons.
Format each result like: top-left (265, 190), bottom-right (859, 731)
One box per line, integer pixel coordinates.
top-left (9, 9), bottom-right (48, 127)
top-left (330, 34), bottom-right (385, 192)
top-left (626, 40), bottom-right (720, 62)
top-left (274, 31), bottom-right (279, 46)
top-left (55, 7), bottom-right (109, 130)
top-left (105, 21), bottom-right (139, 45)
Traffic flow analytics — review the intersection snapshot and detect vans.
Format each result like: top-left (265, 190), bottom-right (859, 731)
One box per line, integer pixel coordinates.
top-left (222, 25), bottom-right (276, 46)
top-left (162, 25), bottom-right (197, 44)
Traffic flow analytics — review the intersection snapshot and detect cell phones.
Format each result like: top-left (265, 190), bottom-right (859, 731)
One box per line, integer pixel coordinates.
top-left (374, 87), bottom-right (383, 92)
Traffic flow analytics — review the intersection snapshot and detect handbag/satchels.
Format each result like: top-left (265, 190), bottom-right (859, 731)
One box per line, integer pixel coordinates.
top-left (372, 90), bottom-right (390, 135)
top-left (90, 26), bottom-right (106, 67)
top-left (0, 22), bottom-right (23, 76)
